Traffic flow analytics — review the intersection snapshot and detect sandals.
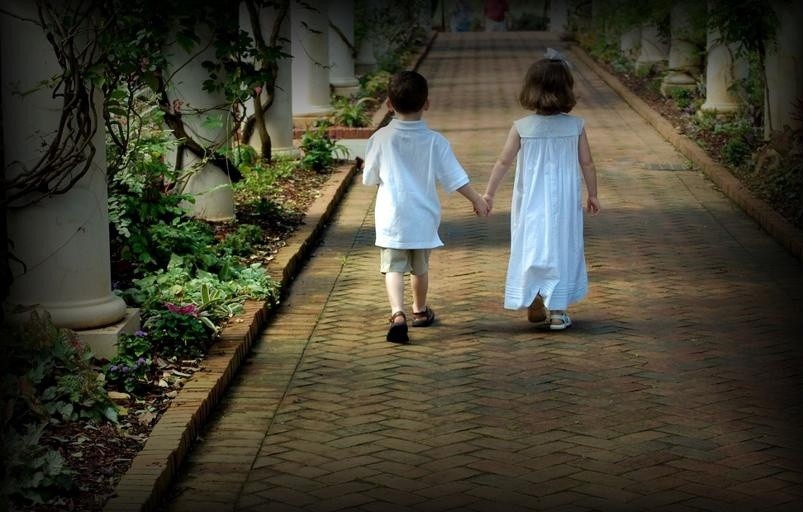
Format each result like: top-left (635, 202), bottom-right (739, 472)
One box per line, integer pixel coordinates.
top-left (529, 296), bottom-right (572, 329)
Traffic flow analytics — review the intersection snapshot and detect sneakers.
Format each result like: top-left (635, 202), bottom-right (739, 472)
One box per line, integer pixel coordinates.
top-left (387, 306), bottom-right (435, 342)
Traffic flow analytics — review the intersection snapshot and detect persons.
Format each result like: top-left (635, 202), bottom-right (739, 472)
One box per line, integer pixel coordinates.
top-left (446, 1), bottom-right (476, 33)
top-left (479, 0), bottom-right (512, 32)
top-left (360, 70), bottom-right (486, 343)
top-left (472, 57), bottom-right (600, 332)
